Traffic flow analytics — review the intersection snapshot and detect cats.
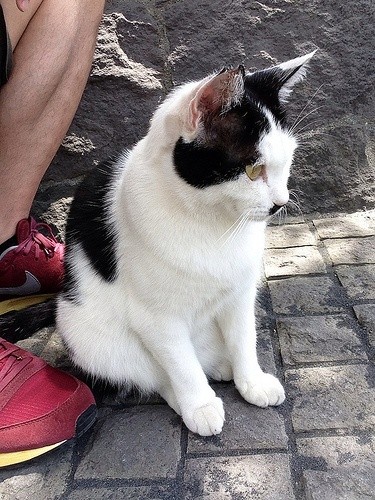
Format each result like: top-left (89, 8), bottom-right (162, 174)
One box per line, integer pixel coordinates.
top-left (54, 50), bottom-right (318, 437)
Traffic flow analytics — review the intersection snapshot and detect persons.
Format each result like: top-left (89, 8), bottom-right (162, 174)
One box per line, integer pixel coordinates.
top-left (0, 1), bottom-right (102, 471)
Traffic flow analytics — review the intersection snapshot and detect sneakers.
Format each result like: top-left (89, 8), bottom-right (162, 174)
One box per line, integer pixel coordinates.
top-left (0, 338), bottom-right (100, 469)
top-left (0, 213), bottom-right (67, 316)
